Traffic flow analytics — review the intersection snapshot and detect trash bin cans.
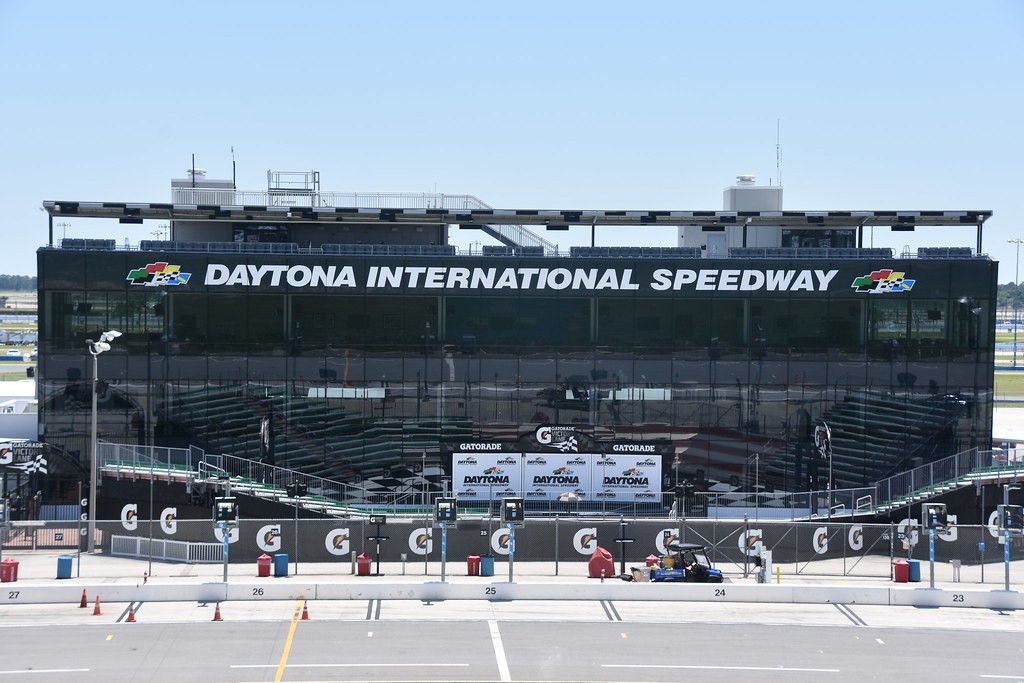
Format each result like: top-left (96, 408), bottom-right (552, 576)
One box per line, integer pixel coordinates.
top-left (891, 560), bottom-right (909, 582)
top-left (466, 555), bottom-right (481, 576)
top-left (481, 554), bottom-right (494, 576)
top-left (907, 560), bottom-right (920, 581)
top-left (0, 557), bottom-right (19, 582)
top-left (357, 552), bottom-right (372, 576)
top-left (56, 555), bottom-right (74, 579)
top-left (645, 553), bottom-right (658, 567)
top-left (274, 554), bottom-right (288, 576)
top-left (257, 554), bottom-right (272, 576)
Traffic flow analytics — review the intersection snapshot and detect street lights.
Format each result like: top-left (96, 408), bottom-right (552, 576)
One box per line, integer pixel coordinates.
top-left (86, 330), bottom-right (122, 554)
top-left (1008, 238), bottom-right (1024, 367)
top-left (158, 224), bottom-right (170, 241)
top-left (150, 230), bottom-right (163, 241)
top-left (57, 222), bottom-right (71, 239)
